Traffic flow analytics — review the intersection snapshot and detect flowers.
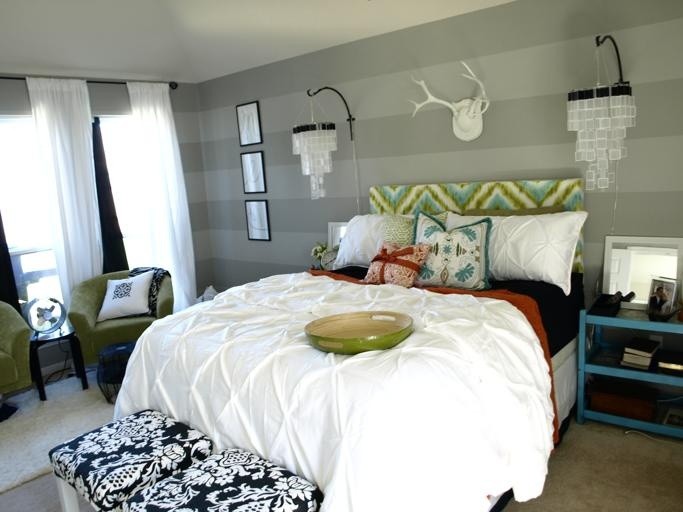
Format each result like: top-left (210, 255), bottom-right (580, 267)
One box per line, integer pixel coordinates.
top-left (310, 242), bottom-right (328, 260)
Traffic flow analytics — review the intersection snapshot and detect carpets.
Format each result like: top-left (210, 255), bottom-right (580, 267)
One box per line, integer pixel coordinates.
top-left (0, 369), bottom-right (115, 495)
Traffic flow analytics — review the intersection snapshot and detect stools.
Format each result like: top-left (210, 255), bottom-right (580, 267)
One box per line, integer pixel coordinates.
top-left (96, 342), bottom-right (136, 404)
top-left (49, 410), bottom-right (214, 511)
top-left (109, 447), bottom-right (321, 512)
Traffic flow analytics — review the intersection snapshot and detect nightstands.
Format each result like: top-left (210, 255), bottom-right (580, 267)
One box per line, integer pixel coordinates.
top-left (577, 308), bottom-right (683, 442)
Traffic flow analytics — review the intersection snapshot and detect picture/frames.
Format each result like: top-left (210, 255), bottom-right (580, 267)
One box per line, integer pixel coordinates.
top-left (646, 279), bottom-right (677, 310)
top-left (235, 99), bottom-right (263, 147)
top-left (243, 199), bottom-right (270, 241)
top-left (240, 151), bottom-right (265, 194)
top-left (327, 221), bottom-right (348, 252)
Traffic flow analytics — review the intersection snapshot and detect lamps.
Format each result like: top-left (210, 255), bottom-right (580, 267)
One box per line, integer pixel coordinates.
top-left (566, 35), bottom-right (638, 234)
top-left (290, 86), bottom-right (361, 218)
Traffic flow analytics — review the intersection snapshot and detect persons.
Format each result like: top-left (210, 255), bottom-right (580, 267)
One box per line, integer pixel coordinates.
top-left (649, 287), bottom-right (664, 312)
top-left (661, 292), bottom-right (671, 313)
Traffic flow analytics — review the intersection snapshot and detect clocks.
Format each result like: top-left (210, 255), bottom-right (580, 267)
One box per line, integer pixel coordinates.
top-left (321, 250), bottom-right (337, 272)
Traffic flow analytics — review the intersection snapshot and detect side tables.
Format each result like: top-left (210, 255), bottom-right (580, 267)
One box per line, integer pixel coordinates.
top-left (29, 319), bottom-right (88, 403)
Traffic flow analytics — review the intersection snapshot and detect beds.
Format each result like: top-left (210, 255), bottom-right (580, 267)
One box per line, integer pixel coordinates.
top-left (115, 179), bottom-right (583, 512)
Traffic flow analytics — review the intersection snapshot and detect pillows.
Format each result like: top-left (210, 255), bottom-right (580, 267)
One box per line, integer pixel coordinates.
top-left (94, 270), bottom-right (156, 323)
top-left (333, 211), bottom-right (587, 296)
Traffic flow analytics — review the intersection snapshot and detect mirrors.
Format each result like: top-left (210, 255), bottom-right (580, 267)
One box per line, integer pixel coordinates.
top-left (602, 236), bottom-right (683, 312)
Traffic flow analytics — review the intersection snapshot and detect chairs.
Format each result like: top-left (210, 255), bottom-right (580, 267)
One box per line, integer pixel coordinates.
top-left (69, 266), bottom-right (173, 374)
top-left (1, 301), bottom-right (29, 403)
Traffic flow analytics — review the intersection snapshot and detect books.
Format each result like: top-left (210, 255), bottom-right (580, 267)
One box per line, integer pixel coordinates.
top-left (658, 349), bottom-right (683, 372)
top-left (619, 335), bottom-right (661, 371)
